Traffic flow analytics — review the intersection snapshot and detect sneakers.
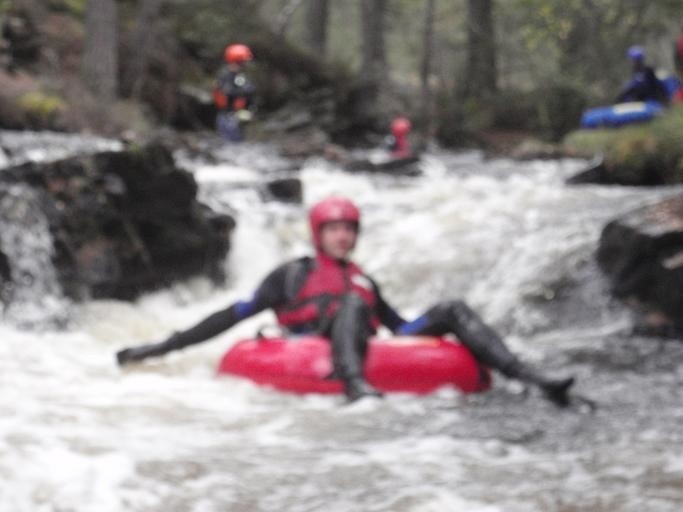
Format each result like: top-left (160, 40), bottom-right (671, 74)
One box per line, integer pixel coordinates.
top-left (540, 377), bottom-right (574, 396)
top-left (346, 380), bottom-right (379, 400)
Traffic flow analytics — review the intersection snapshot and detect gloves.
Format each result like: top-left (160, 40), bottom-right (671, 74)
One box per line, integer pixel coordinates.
top-left (116, 345), bottom-right (151, 365)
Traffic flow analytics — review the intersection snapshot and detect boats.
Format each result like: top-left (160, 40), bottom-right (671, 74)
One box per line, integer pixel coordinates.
top-left (335, 145), bottom-right (425, 177)
top-left (213, 110), bottom-right (317, 145)
top-left (217, 329), bottom-right (504, 406)
top-left (572, 97), bottom-right (666, 130)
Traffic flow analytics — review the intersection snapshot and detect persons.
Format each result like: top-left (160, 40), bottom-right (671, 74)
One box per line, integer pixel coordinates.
top-left (615, 45), bottom-right (667, 105)
top-left (116, 196), bottom-right (579, 405)
top-left (214, 44), bottom-right (255, 144)
top-left (389, 117), bottom-right (413, 158)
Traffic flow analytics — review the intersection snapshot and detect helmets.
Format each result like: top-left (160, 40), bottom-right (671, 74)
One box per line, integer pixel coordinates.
top-left (311, 198), bottom-right (359, 249)
top-left (224, 43), bottom-right (254, 64)
